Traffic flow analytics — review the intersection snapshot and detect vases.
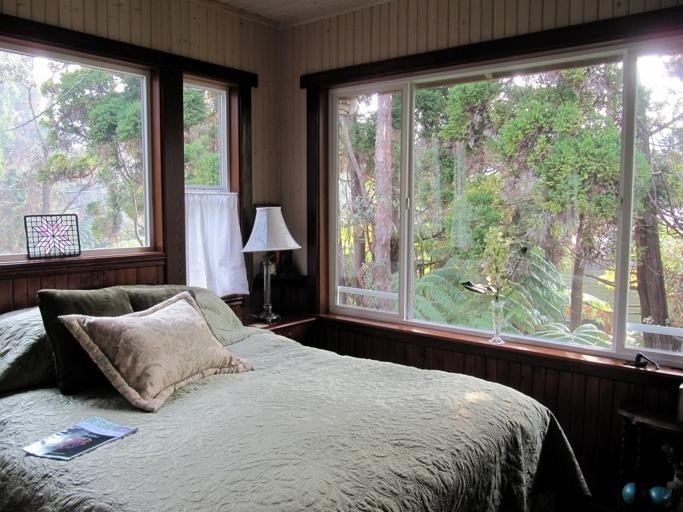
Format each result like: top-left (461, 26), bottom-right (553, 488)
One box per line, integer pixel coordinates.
top-left (488, 299), bottom-right (505, 345)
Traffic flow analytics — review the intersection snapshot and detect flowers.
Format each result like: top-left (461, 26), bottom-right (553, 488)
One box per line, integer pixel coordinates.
top-left (456, 222), bottom-right (530, 336)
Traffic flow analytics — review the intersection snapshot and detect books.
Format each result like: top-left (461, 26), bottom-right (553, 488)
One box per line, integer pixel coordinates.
top-left (23, 416), bottom-right (138, 461)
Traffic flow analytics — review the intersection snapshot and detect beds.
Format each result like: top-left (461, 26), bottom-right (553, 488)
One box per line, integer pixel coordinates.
top-left (0, 314), bottom-right (557, 512)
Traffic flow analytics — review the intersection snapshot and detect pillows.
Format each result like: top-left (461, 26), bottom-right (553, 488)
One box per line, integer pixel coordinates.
top-left (34, 286), bottom-right (257, 413)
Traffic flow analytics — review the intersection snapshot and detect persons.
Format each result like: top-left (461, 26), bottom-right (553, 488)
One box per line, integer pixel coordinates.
top-left (45, 436), bottom-right (91, 453)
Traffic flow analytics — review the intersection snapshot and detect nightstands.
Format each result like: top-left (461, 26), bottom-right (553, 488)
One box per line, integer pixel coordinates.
top-left (242, 312), bottom-right (317, 356)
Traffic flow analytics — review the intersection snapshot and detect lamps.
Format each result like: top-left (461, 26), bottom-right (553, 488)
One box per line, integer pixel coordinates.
top-left (240, 206), bottom-right (302, 325)
